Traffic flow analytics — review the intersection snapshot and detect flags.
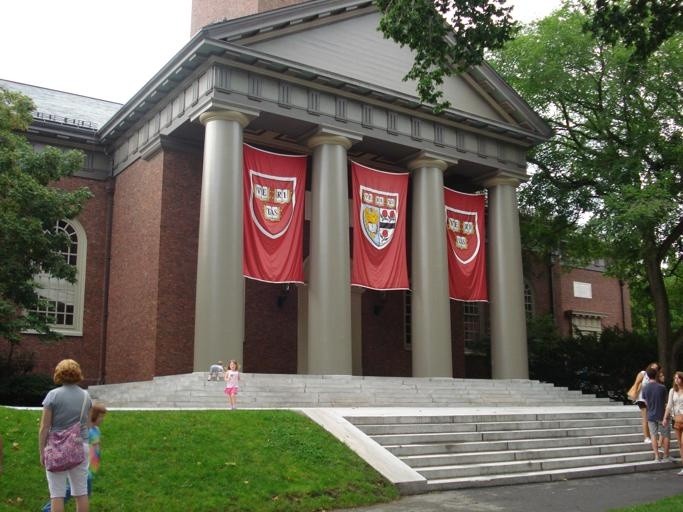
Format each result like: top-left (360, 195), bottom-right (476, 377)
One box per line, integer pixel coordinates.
top-left (443, 185), bottom-right (489, 303)
top-left (351, 158), bottom-right (410, 291)
top-left (243, 142), bottom-right (308, 285)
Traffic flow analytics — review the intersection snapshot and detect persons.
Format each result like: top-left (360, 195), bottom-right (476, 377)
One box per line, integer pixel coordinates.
top-left (207, 359), bottom-right (241, 410)
top-left (39, 358), bottom-right (106, 512)
top-left (627, 361), bottom-right (683, 476)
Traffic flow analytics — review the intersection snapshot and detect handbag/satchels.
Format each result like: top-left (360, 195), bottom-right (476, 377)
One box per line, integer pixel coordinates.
top-left (41, 421), bottom-right (86, 474)
top-left (673, 415), bottom-right (683, 430)
top-left (626, 382), bottom-right (642, 401)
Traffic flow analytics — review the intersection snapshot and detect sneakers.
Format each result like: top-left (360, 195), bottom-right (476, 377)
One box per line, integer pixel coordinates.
top-left (644, 437), bottom-right (651, 444)
top-left (677, 470), bottom-right (683, 476)
top-left (654, 455), bottom-right (678, 463)
top-left (207, 374), bottom-right (212, 381)
top-left (217, 375), bottom-right (219, 381)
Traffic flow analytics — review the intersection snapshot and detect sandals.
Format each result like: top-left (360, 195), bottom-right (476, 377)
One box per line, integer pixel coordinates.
top-left (228, 406), bottom-right (235, 409)
